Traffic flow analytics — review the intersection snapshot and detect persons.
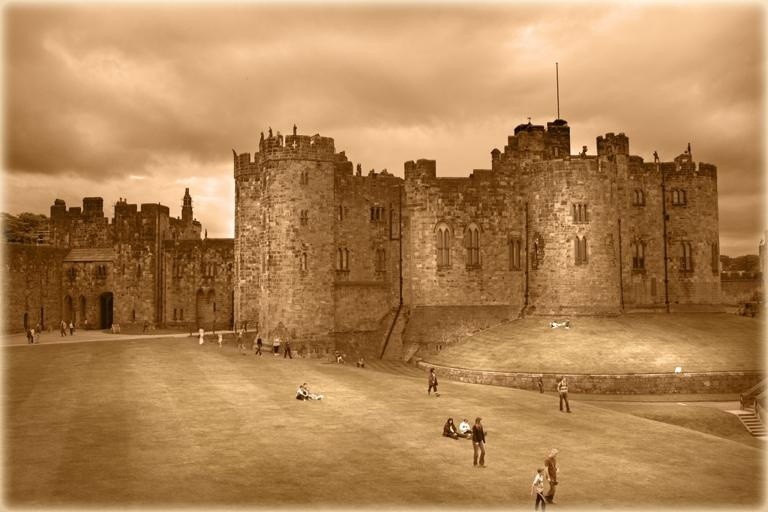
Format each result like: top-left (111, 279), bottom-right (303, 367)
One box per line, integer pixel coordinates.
top-left (459, 419), bottom-right (487, 435)
top-left (304, 383), bottom-right (312, 399)
top-left (443, 418), bottom-right (468, 440)
top-left (544, 449), bottom-right (559, 504)
top-left (198, 327), bottom-right (293, 359)
top-left (260, 124), bottom-right (297, 140)
top-left (688, 143), bottom-right (690, 154)
top-left (357, 359), bottom-right (363, 366)
top-left (654, 151), bottom-right (660, 164)
top-left (531, 468), bottom-right (546, 511)
top-left (538, 377), bottom-right (544, 394)
top-left (428, 368), bottom-right (440, 397)
top-left (471, 417), bottom-right (486, 468)
top-left (143, 320), bottom-right (149, 332)
top-left (557, 376), bottom-right (572, 413)
top-left (296, 385), bottom-right (309, 401)
top-left (26, 320), bottom-right (75, 343)
